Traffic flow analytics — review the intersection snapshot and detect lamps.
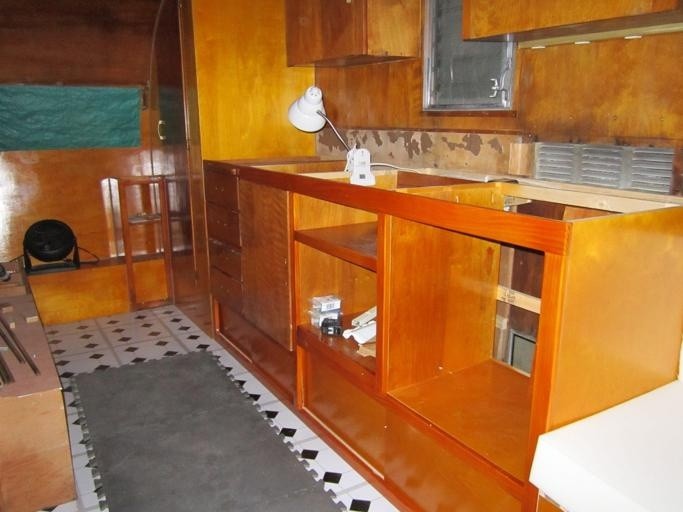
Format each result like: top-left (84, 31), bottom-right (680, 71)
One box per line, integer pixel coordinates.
top-left (287, 86), bottom-right (375, 187)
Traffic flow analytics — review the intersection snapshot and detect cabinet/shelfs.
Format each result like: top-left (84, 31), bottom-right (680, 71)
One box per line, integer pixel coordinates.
top-left (462, 1), bottom-right (683, 42)
top-left (118, 174), bottom-right (175, 310)
top-left (202, 154), bottom-right (683, 512)
top-left (283, 0), bottom-right (421, 70)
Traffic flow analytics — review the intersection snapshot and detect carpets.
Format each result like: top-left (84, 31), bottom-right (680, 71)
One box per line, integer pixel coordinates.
top-left (70, 350), bottom-right (344, 512)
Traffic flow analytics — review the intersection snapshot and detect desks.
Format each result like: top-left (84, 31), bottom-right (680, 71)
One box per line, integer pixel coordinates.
top-left (529, 378), bottom-right (683, 512)
